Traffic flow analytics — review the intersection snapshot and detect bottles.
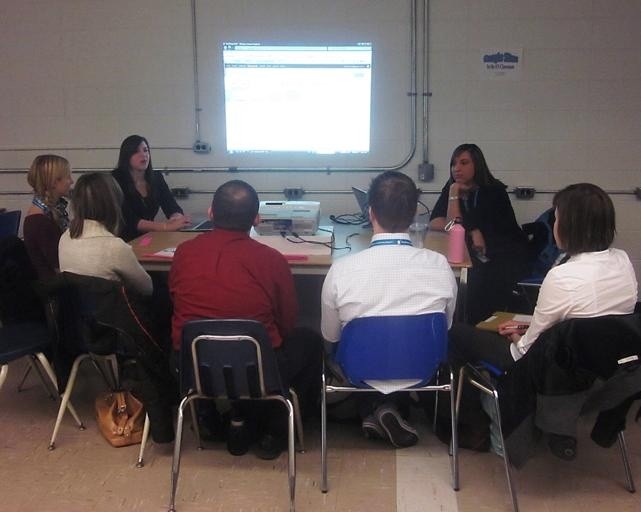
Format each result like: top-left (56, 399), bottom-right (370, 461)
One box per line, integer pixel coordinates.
top-left (447, 216), bottom-right (465, 264)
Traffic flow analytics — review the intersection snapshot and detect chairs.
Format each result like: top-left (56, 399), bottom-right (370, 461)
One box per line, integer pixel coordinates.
top-left (0, 209), bottom-right (58, 403)
top-left (316, 312), bottom-right (467, 494)
top-left (519, 205), bottom-right (562, 306)
top-left (464, 313), bottom-right (641, 511)
top-left (157, 319), bottom-right (307, 511)
top-left (46, 272), bottom-right (153, 467)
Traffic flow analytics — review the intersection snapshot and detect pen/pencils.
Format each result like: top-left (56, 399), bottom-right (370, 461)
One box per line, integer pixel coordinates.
top-left (505, 325), bottom-right (529, 329)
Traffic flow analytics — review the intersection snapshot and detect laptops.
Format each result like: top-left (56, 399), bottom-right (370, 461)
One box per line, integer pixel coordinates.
top-left (351, 186), bottom-right (367, 214)
top-left (179, 219), bottom-right (214, 232)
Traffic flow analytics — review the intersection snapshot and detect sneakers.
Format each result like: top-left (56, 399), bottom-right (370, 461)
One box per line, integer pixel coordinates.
top-left (257, 436), bottom-right (287, 459)
top-left (226, 425), bottom-right (249, 455)
top-left (362, 415), bottom-right (385, 440)
top-left (436, 421), bottom-right (491, 452)
top-left (375, 407), bottom-right (419, 449)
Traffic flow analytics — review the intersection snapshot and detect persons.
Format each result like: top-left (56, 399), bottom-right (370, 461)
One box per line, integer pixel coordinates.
top-left (58, 172), bottom-right (154, 297)
top-left (111, 135), bottom-right (193, 235)
top-left (24, 155), bottom-right (74, 315)
top-left (461, 183), bottom-right (638, 373)
top-left (428, 144), bottom-right (529, 309)
top-left (322, 170), bottom-right (459, 446)
top-left (167, 180), bottom-right (322, 446)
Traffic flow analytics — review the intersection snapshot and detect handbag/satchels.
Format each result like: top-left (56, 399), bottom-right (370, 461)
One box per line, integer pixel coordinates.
top-left (93, 388), bottom-right (145, 448)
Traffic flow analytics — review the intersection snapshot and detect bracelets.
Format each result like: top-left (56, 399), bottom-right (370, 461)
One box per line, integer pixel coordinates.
top-left (448, 196), bottom-right (460, 200)
top-left (160, 223), bottom-right (167, 231)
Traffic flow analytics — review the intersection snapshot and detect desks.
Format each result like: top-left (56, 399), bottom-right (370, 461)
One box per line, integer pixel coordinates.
top-left (116, 214), bottom-right (474, 349)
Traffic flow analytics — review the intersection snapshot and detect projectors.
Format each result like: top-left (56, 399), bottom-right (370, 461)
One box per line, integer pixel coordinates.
top-left (253, 200), bottom-right (320, 236)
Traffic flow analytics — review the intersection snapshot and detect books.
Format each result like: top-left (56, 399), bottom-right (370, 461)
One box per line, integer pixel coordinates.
top-left (475, 311), bottom-right (534, 333)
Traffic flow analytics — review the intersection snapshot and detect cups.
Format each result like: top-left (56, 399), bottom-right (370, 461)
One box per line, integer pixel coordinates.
top-left (409, 223), bottom-right (429, 248)
